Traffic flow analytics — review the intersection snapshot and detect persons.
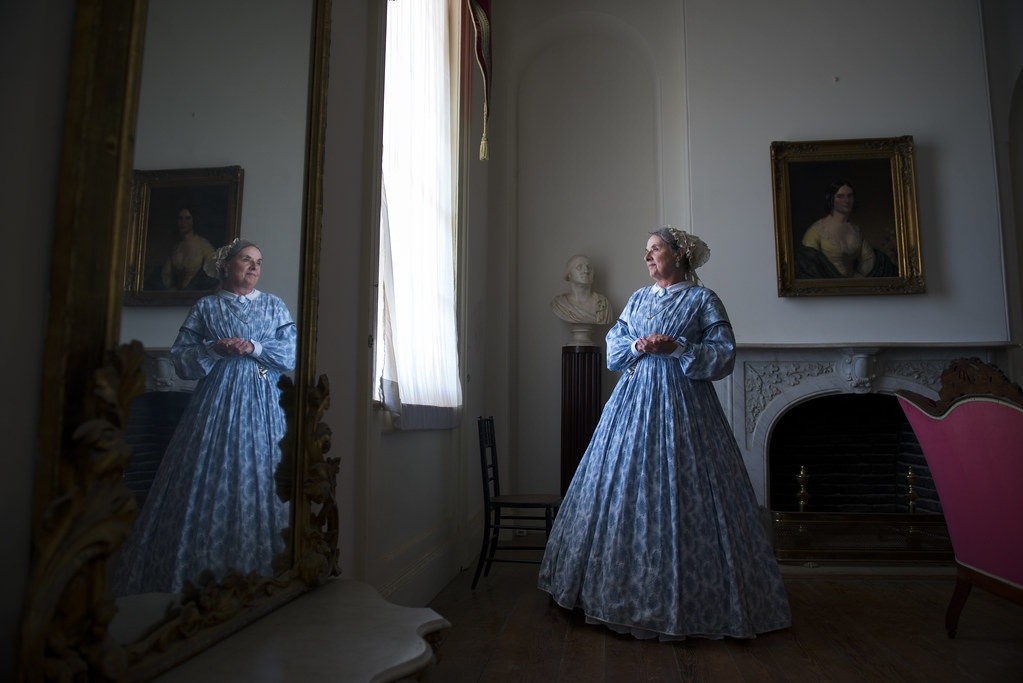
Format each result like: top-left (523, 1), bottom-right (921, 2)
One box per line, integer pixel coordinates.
top-left (117, 240), bottom-right (299, 618)
top-left (534, 224), bottom-right (794, 637)
top-left (549, 253), bottom-right (615, 325)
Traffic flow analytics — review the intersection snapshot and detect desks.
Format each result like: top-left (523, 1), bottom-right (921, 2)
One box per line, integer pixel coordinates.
top-left (150, 579), bottom-right (453, 683)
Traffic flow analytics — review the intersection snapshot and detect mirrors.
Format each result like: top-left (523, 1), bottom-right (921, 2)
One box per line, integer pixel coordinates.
top-left (21, 0), bottom-right (345, 683)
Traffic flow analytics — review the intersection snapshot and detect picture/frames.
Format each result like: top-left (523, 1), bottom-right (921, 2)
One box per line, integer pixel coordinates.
top-left (122, 165), bottom-right (245, 308)
top-left (769, 135), bottom-right (925, 297)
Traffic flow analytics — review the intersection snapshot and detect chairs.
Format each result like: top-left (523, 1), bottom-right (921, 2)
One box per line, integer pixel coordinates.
top-left (470, 415), bottom-right (563, 590)
top-left (893, 358), bottom-right (1023, 641)
